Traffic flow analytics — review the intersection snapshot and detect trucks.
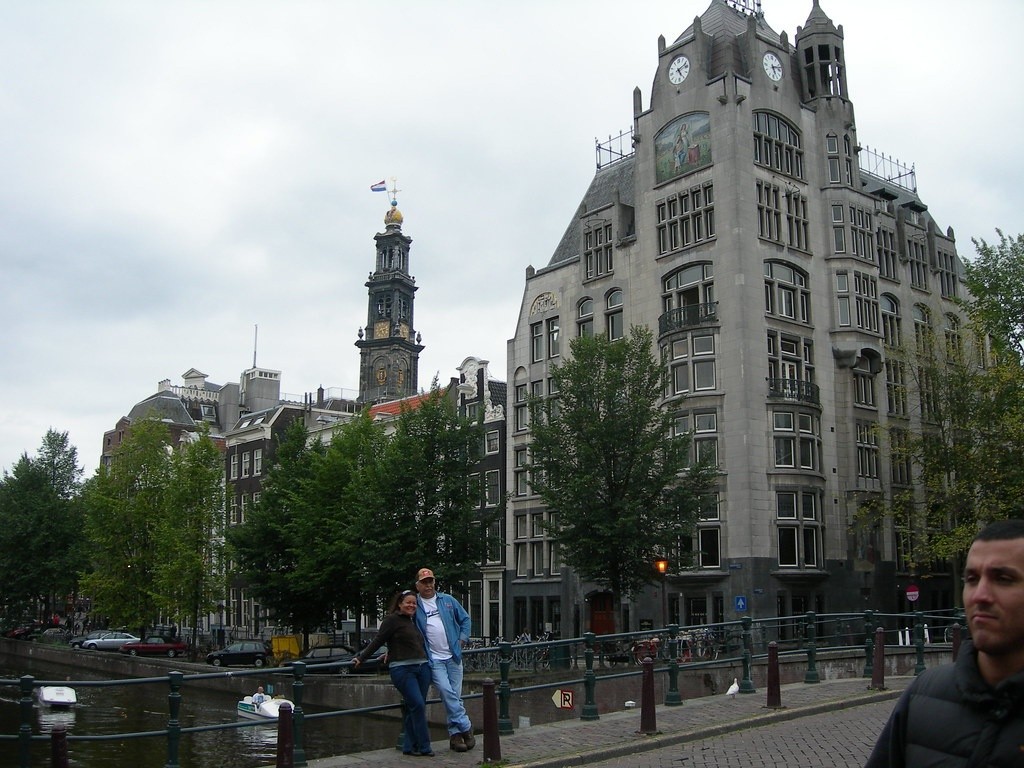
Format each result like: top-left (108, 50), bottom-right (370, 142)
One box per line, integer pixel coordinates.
top-left (271, 634), bottom-right (328, 664)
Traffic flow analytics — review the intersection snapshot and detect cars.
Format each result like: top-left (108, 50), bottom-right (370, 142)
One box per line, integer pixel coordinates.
top-left (82, 632), bottom-right (140, 650)
top-left (119, 635), bottom-right (190, 659)
top-left (205, 641), bottom-right (273, 668)
top-left (69, 629), bottom-right (110, 648)
top-left (277, 646), bottom-right (392, 679)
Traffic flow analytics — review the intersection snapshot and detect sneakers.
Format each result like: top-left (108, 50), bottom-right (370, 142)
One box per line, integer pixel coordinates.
top-left (463, 724), bottom-right (476, 749)
top-left (450, 734), bottom-right (468, 752)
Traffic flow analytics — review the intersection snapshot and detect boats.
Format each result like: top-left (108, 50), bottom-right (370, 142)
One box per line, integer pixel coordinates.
top-left (36, 685), bottom-right (77, 705)
top-left (237, 694), bottom-right (295, 723)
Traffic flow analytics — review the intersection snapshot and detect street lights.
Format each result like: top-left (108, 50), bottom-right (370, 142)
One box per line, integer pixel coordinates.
top-left (654, 556), bottom-right (671, 624)
top-left (217, 603), bottom-right (225, 649)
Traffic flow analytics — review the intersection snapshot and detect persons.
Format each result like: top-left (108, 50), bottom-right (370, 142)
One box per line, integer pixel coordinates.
top-left (377, 567), bottom-right (476, 751)
top-left (864, 521), bottom-right (1024, 768)
top-left (351, 590), bottom-right (436, 757)
top-left (51, 612), bottom-right (90, 635)
top-left (251, 686), bottom-right (268, 708)
top-left (518, 628), bottom-right (532, 644)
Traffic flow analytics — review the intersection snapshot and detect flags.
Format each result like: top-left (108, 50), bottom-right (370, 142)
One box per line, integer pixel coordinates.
top-left (370, 180), bottom-right (386, 192)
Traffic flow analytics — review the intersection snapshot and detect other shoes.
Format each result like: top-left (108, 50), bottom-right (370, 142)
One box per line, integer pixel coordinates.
top-left (403, 749), bottom-right (435, 757)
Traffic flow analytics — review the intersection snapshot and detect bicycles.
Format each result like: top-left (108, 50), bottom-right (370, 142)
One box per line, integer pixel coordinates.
top-left (461, 629), bottom-right (726, 674)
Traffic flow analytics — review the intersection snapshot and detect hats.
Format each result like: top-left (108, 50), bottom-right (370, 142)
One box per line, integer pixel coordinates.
top-left (417, 568), bottom-right (437, 580)
